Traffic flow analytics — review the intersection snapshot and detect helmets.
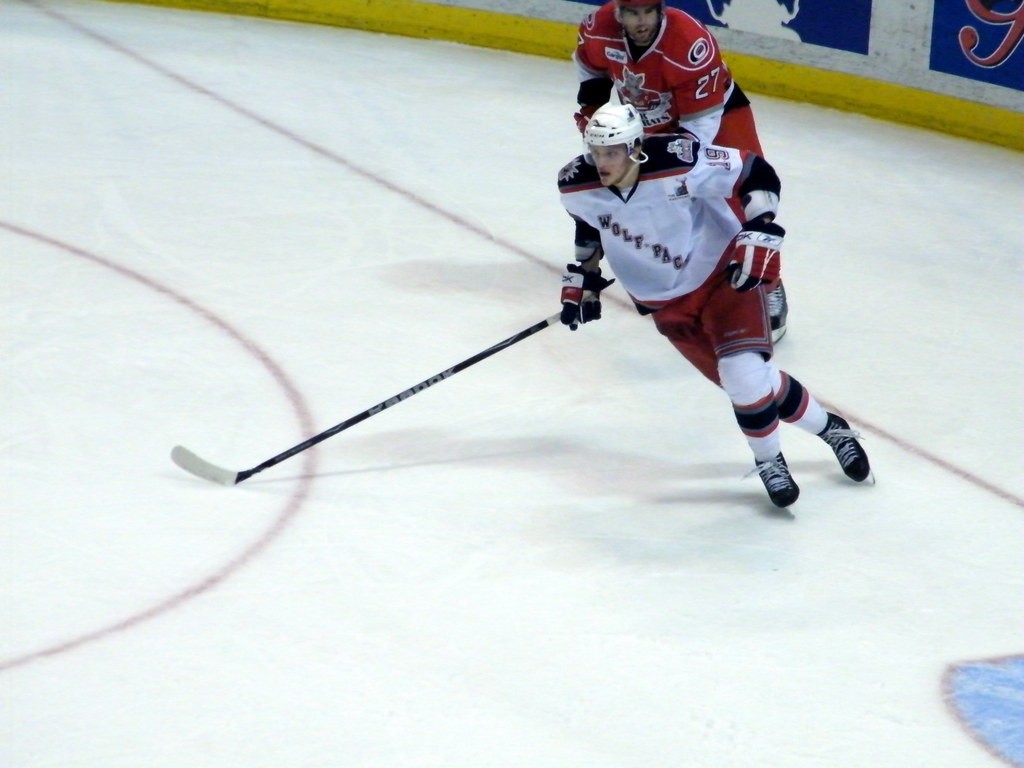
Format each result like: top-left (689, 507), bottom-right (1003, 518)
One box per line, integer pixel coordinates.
top-left (614, 0), bottom-right (660, 23)
top-left (582, 102), bottom-right (645, 154)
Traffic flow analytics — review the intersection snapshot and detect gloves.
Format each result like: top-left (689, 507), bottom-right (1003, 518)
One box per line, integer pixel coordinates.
top-left (561, 264), bottom-right (616, 331)
top-left (722, 218), bottom-right (786, 292)
top-left (574, 105), bottom-right (601, 134)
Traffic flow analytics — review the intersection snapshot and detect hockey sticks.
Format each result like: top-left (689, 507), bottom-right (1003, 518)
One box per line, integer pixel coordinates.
top-left (168, 293), bottom-right (601, 490)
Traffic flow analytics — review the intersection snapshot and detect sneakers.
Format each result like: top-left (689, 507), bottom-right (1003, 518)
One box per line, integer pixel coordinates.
top-left (815, 411), bottom-right (876, 484)
top-left (746, 451), bottom-right (800, 517)
top-left (764, 278), bottom-right (788, 345)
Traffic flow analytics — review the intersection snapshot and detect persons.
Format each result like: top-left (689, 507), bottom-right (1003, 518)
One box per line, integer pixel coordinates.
top-left (572, 0), bottom-right (788, 330)
top-left (557, 104), bottom-right (871, 509)
